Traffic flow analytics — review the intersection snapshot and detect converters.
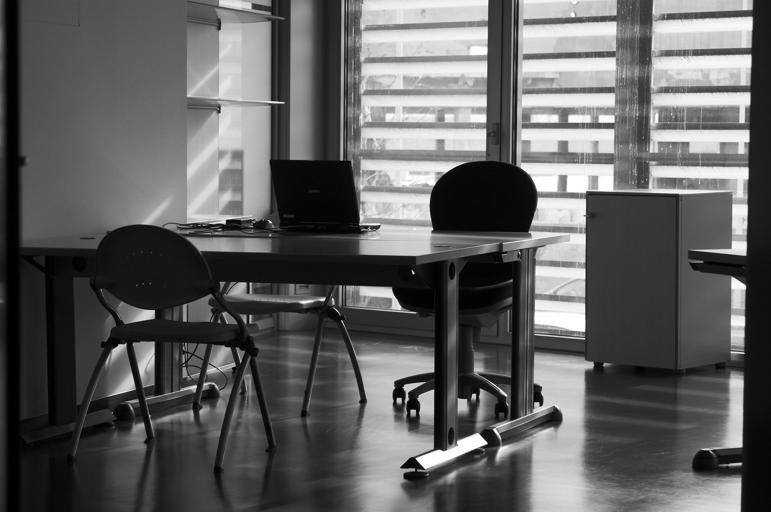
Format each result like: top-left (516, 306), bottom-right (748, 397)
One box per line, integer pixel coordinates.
top-left (226, 217), bottom-right (256, 228)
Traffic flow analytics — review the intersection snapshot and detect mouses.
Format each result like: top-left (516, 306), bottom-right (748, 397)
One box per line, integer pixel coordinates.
top-left (253, 218), bottom-right (273, 230)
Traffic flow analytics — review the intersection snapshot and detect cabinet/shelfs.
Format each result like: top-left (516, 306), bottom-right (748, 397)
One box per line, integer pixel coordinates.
top-left (584, 189), bottom-right (733, 377)
top-left (187, 0), bottom-right (285, 225)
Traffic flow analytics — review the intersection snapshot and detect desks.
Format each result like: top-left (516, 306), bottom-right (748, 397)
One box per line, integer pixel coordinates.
top-left (686, 248), bottom-right (747, 470)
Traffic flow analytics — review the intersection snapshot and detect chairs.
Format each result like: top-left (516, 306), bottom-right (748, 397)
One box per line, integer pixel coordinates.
top-left (392, 161), bottom-right (543, 421)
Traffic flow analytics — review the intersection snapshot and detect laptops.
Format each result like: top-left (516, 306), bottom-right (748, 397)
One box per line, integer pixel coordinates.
top-left (269, 159), bottom-right (381, 234)
